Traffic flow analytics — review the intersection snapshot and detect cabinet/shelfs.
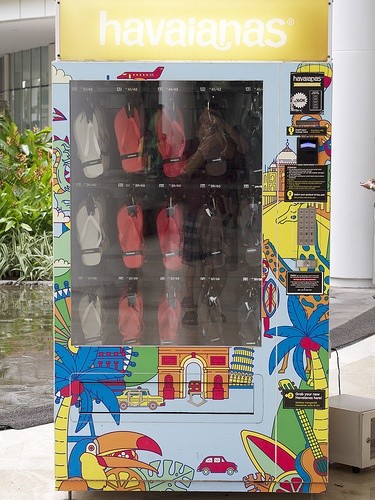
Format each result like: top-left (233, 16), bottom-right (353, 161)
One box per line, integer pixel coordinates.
top-left (46, 2), bottom-right (333, 495)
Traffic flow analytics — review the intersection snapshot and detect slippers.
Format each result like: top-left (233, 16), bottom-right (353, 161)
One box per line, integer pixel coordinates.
top-left (72, 99), bottom-right (261, 346)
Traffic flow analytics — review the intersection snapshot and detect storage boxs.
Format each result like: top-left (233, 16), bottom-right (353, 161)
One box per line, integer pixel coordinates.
top-left (328, 393), bottom-right (375, 468)
